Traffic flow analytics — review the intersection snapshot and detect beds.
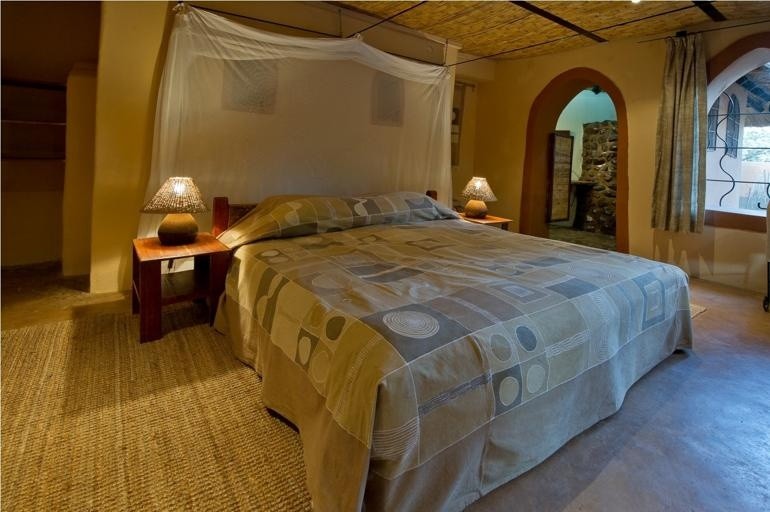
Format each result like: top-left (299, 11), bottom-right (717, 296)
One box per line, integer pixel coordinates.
top-left (210, 190), bottom-right (690, 512)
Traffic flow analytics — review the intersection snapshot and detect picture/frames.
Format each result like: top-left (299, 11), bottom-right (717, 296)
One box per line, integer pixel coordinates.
top-left (370, 72), bottom-right (405, 126)
top-left (221, 43), bottom-right (277, 116)
top-left (546, 132), bottom-right (574, 223)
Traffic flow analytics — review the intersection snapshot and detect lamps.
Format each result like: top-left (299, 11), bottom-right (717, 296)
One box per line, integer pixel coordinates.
top-left (140, 176), bottom-right (209, 246)
top-left (459, 177), bottom-right (498, 219)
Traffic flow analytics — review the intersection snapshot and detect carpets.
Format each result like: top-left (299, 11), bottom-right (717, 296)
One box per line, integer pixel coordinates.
top-left (0, 301), bottom-right (319, 511)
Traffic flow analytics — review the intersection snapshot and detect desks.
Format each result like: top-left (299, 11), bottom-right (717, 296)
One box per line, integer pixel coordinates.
top-left (129, 231), bottom-right (232, 343)
top-left (458, 212), bottom-right (514, 231)
